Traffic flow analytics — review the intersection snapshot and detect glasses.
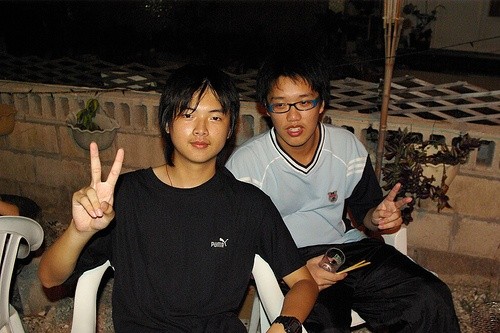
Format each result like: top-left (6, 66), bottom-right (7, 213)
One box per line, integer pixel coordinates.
top-left (265, 95), bottom-right (323, 114)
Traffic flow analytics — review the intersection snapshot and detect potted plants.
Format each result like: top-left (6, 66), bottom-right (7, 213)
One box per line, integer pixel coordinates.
top-left (403, 3), bottom-right (446, 51)
top-left (64, 100), bottom-right (119, 151)
top-left (367, 125), bottom-right (482, 226)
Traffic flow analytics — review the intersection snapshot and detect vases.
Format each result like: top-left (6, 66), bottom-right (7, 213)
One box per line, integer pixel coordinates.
top-left (0, 103), bottom-right (17, 135)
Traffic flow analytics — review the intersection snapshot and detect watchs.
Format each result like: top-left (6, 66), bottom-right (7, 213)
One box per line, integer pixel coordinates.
top-left (275, 315), bottom-right (302, 333)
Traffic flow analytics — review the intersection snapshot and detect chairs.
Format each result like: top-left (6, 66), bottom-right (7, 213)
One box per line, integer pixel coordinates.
top-left (71, 253), bottom-right (307, 333)
top-left (0, 215), bottom-right (44, 333)
top-left (249, 227), bottom-right (438, 333)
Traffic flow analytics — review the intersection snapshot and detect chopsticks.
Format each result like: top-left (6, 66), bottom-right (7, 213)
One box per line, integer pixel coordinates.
top-left (336, 260), bottom-right (370, 274)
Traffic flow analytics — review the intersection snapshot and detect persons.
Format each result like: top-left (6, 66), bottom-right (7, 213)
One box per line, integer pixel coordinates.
top-left (224, 43), bottom-right (461, 333)
top-left (37, 65), bottom-right (318, 333)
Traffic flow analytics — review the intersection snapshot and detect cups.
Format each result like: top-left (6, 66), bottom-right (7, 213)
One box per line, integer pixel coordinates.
top-left (318, 248), bottom-right (345, 272)
